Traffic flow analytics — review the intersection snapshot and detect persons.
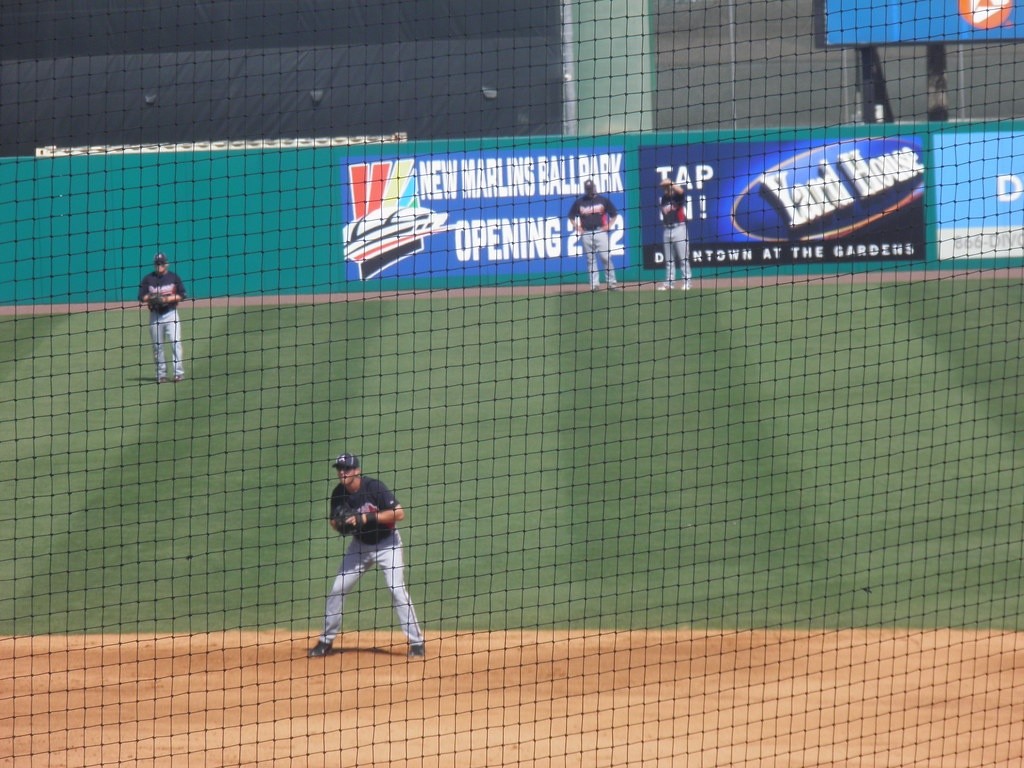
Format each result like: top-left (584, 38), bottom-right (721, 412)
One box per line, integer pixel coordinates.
top-left (308, 451), bottom-right (424, 657)
top-left (138, 253), bottom-right (185, 383)
top-left (657, 178), bottom-right (692, 290)
top-left (569, 179), bottom-right (618, 291)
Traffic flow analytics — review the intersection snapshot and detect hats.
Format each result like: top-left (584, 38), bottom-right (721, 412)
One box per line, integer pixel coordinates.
top-left (155, 254), bottom-right (166, 263)
top-left (332, 452), bottom-right (358, 470)
top-left (584, 180), bottom-right (595, 189)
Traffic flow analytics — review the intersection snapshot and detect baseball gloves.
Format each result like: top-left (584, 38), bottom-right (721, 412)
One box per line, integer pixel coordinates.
top-left (148, 293), bottom-right (169, 312)
top-left (336, 512), bottom-right (377, 535)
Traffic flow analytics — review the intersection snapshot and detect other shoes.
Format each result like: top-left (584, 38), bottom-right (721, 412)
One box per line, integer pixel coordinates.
top-left (682, 279), bottom-right (691, 290)
top-left (159, 377), bottom-right (166, 382)
top-left (658, 281), bottom-right (674, 290)
top-left (172, 375), bottom-right (181, 381)
top-left (605, 285), bottom-right (620, 291)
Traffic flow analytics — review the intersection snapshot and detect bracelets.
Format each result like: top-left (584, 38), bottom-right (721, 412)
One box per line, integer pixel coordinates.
top-left (670, 182), bottom-right (674, 186)
top-left (364, 512), bottom-right (376, 526)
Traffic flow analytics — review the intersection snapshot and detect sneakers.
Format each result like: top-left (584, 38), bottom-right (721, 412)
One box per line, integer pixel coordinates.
top-left (410, 644), bottom-right (424, 656)
top-left (309, 643), bottom-right (331, 657)
